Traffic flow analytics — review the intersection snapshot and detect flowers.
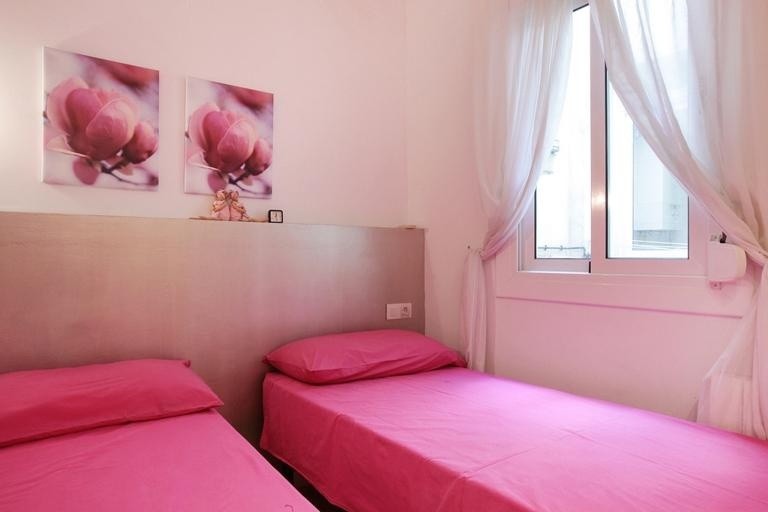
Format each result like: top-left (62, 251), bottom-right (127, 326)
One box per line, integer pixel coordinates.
top-left (44, 75), bottom-right (159, 189)
top-left (186, 100), bottom-right (273, 197)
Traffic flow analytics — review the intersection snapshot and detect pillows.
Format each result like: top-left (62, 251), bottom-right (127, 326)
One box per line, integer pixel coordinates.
top-left (2, 358), bottom-right (225, 446)
top-left (2, 408), bottom-right (320, 511)
top-left (264, 330), bottom-right (467, 385)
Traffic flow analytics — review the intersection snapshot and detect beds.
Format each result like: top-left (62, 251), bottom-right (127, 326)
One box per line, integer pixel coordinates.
top-left (259, 369), bottom-right (768, 510)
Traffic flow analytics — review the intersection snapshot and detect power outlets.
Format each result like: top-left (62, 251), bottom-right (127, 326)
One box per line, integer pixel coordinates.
top-left (401, 303), bottom-right (412, 319)
